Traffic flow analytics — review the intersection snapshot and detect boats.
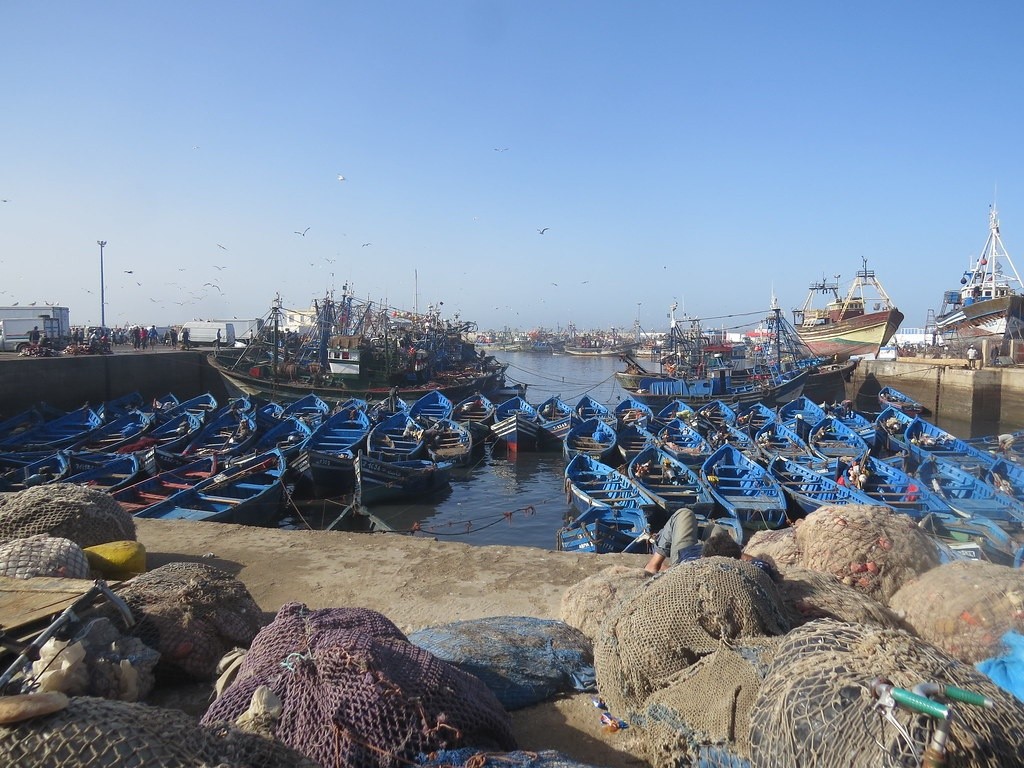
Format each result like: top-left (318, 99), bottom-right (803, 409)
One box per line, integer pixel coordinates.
top-left (562, 415), bottom-right (617, 462)
top-left (808, 412), bottom-right (869, 465)
top-left (537, 394), bottom-right (575, 438)
top-left (755, 418), bottom-right (814, 463)
top-left (0, 405), bottom-right (44, 444)
top-left (778, 393), bottom-right (828, 443)
top-left (656, 398), bottom-right (696, 430)
top-left (490, 413), bottom-right (558, 454)
top-left (842, 450), bottom-right (952, 521)
top-left (107, 452), bottom-right (219, 515)
top-left (916, 510), bottom-right (1024, 571)
top-left (735, 401), bottom-right (777, 440)
top-left (556, 506), bottom-right (650, 556)
top-left (299, 402), bottom-right (373, 476)
top-left (207, 269), bottom-right (509, 406)
top-left (627, 443), bottom-right (715, 521)
top-left (982, 440), bottom-right (1024, 472)
top-left (573, 391), bottom-right (609, 423)
top-left (365, 409), bottom-right (425, 463)
top-left (449, 389), bottom-right (495, 439)
top-left (905, 414), bottom-right (995, 482)
top-left (767, 452), bottom-right (890, 522)
top-left (697, 398), bottom-right (736, 438)
top-left (353, 448), bottom-right (435, 507)
top-left (699, 440), bottom-right (788, 531)
top-left (877, 384), bottom-right (924, 419)
top-left (493, 394), bottom-right (537, 425)
top-left (984, 455), bottom-right (1024, 506)
top-left (874, 404), bottom-right (914, 454)
top-left (743, 254), bottom-right (906, 359)
top-left (368, 393), bottom-right (411, 425)
top-left (656, 417), bottom-right (716, 465)
top-left (611, 395), bottom-right (654, 429)
top-left (565, 452), bottom-right (656, 518)
top-left (934, 181), bottom-right (1024, 360)
top-left (616, 421), bottom-right (662, 463)
top-left (424, 416), bottom-right (474, 469)
top-left (818, 399), bottom-right (871, 437)
top-left (650, 513), bottom-right (745, 560)
top-left (913, 452), bottom-right (1024, 547)
top-left (0, 393), bottom-right (366, 492)
top-left (133, 447), bottom-right (289, 528)
top-left (476, 296), bottom-right (862, 416)
top-left (709, 422), bottom-right (762, 464)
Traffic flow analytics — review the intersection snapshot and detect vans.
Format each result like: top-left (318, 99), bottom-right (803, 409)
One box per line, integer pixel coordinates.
top-left (177, 321), bottom-right (235, 348)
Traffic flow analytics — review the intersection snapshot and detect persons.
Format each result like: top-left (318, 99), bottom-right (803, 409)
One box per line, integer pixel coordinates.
top-left (966, 344), bottom-right (978, 370)
top-left (70, 325), bottom-right (192, 351)
top-left (642, 508), bottom-right (772, 576)
top-left (29, 325), bottom-right (52, 351)
top-left (250, 329), bottom-right (253, 343)
top-left (217, 328), bottom-right (222, 350)
top-left (990, 346), bottom-right (999, 367)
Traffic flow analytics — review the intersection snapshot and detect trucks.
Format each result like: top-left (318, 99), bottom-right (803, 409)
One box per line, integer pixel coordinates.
top-left (0, 307), bottom-right (69, 353)
top-left (203, 318), bottom-right (264, 343)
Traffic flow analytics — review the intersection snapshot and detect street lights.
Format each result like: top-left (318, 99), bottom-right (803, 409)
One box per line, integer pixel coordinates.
top-left (97, 239), bottom-right (107, 326)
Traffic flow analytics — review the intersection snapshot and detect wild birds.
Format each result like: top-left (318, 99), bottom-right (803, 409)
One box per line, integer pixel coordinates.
top-left (193, 318), bottom-right (214, 322)
top-left (233, 317), bottom-right (237, 319)
top-left (124, 270), bottom-right (133, 274)
top-left (294, 227), bottom-right (310, 237)
top-left (137, 282), bottom-right (142, 287)
top-left (537, 228), bottom-right (549, 235)
top-left (218, 244), bottom-right (226, 250)
top-left (552, 283), bottom-right (557, 287)
top-left (338, 174), bottom-right (345, 181)
top-left (169, 266), bottom-right (226, 305)
top-left (362, 243), bottom-right (371, 248)
top-left (326, 259), bottom-right (335, 264)
top-left (0, 290), bottom-right (59, 306)
